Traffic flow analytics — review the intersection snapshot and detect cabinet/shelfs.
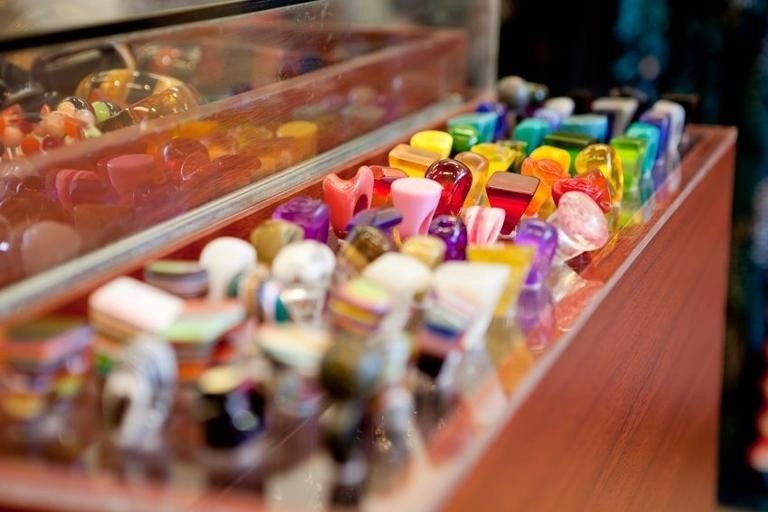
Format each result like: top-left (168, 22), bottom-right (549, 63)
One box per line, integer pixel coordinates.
top-left (0, 1), bottom-right (739, 511)
